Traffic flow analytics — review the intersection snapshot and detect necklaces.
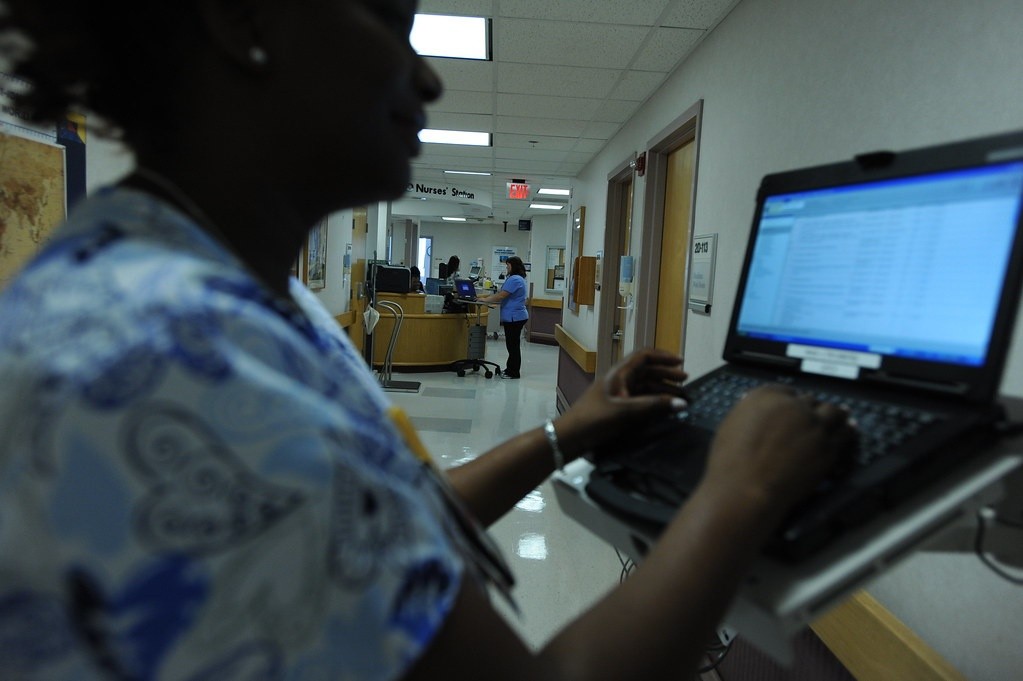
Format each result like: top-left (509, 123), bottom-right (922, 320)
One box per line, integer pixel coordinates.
top-left (134, 160), bottom-right (252, 271)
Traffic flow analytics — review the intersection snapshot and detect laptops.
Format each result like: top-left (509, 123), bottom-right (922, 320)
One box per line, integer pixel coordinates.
top-left (455, 280), bottom-right (477, 301)
top-left (468, 266), bottom-right (482, 279)
top-left (584, 129), bottom-right (1023, 566)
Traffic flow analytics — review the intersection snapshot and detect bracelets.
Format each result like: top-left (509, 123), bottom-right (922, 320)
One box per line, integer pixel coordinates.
top-left (543, 418), bottom-right (563, 471)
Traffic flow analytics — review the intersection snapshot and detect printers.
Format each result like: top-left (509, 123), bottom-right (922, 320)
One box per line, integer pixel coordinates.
top-left (374, 264), bottom-right (411, 293)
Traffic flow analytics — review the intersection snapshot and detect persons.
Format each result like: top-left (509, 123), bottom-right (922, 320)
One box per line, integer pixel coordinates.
top-left (472, 256), bottom-right (531, 379)
top-left (1, 2), bottom-right (859, 681)
top-left (412, 266), bottom-right (426, 292)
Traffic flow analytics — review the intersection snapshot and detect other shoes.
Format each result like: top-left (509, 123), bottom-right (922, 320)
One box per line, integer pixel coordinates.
top-left (501, 373), bottom-right (519, 379)
top-left (501, 369), bottom-right (508, 374)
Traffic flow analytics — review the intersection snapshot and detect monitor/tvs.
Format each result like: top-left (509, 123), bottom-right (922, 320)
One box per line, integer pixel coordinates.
top-left (438, 285), bottom-right (454, 295)
